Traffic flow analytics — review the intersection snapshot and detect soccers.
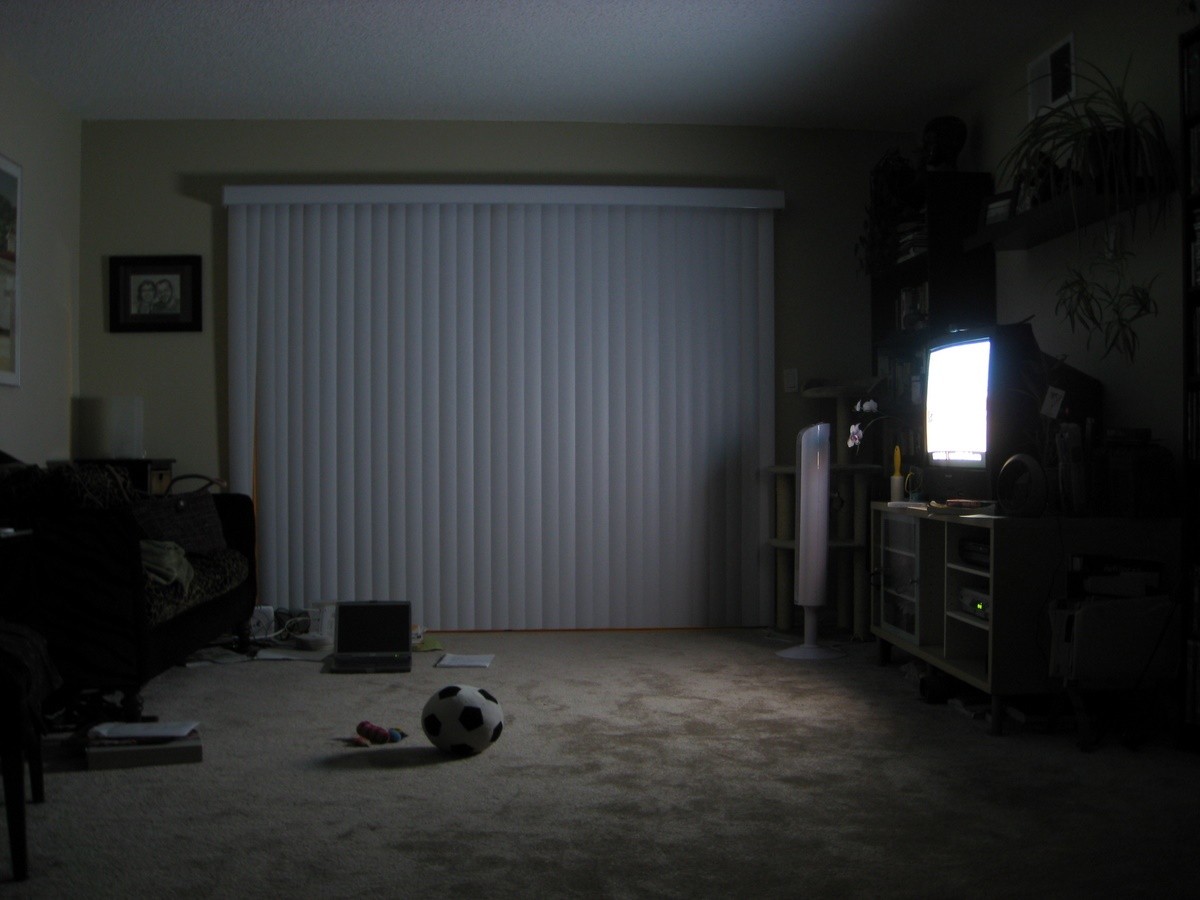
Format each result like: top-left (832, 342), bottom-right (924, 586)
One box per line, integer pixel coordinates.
top-left (422, 684), bottom-right (504, 755)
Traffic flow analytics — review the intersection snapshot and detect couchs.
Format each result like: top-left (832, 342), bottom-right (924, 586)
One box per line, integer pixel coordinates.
top-left (0, 452), bottom-right (256, 725)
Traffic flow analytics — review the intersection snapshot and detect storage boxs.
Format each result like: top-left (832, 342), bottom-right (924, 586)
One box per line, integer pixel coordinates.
top-left (85, 726), bottom-right (202, 771)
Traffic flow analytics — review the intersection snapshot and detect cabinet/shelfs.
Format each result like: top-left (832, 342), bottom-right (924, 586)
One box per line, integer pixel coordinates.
top-left (870, 500), bottom-right (1180, 734)
top-left (871, 171), bottom-right (995, 501)
top-left (768, 383), bottom-right (884, 641)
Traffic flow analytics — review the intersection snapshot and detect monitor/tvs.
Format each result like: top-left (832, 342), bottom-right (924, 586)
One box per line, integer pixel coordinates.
top-left (921, 320), bottom-right (1107, 502)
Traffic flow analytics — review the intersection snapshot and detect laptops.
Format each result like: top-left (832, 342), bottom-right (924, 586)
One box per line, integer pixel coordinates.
top-left (319, 600), bottom-right (412, 673)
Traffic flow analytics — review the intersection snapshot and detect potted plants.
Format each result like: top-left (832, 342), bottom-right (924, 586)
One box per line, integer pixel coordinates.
top-left (979, 55), bottom-right (1175, 251)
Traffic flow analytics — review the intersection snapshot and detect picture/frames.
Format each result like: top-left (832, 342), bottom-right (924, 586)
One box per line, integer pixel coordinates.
top-left (108, 254), bottom-right (202, 333)
top-left (0, 157), bottom-right (20, 389)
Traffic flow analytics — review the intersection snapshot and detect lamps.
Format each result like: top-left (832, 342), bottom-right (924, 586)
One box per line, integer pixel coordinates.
top-left (69, 396), bottom-right (145, 462)
top-left (776, 421), bottom-right (835, 661)
